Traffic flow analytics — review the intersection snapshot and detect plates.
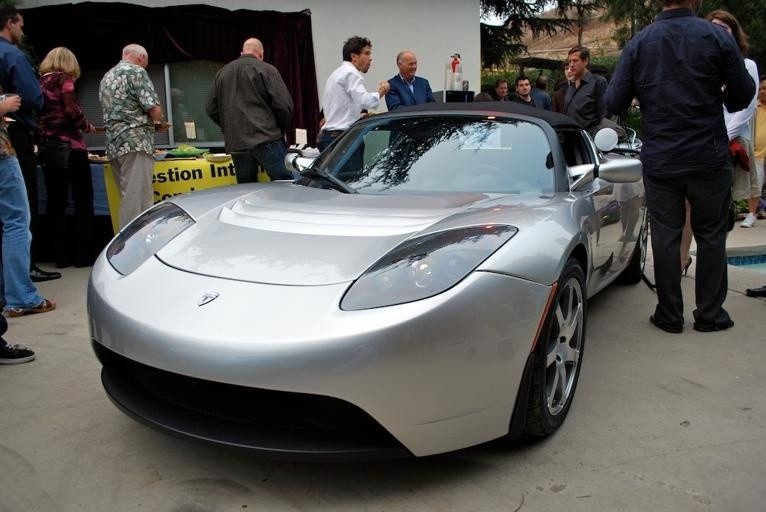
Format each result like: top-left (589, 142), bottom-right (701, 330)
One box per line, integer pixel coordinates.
top-left (152, 148), bottom-right (232, 162)
top-left (91, 126), bottom-right (106, 134)
top-left (154, 122), bottom-right (173, 134)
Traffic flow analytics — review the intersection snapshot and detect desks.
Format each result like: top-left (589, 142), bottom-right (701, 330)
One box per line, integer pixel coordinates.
top-left (90, 157), bottom-right (271, 236)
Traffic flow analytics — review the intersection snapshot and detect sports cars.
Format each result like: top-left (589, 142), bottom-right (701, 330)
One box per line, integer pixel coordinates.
top-left (81, 96), bottom-right (650, 463)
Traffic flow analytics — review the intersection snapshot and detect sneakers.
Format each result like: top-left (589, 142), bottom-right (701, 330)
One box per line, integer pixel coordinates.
top-left (0, 338), bottom-right (35, 365)
top-left (5, 297), bottom-right (59, 317)
top-left (739, 213), bottom-right (757, 229)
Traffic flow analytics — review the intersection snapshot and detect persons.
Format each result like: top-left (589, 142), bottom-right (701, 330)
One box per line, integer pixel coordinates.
top-left (680, 10), bottom-right (761, 275)
top-left (203, 36), bottom-right (296, 184)
top-left (170, 85), bottom-right (200, 142)
top-left (386, 52), bottom-right (437, 147)
top-left (1, 92), bottom-right (57, 318)
top-left (599, 0), bottom-right (757, 333)
top-left (316, 36), bottom-right (392, 172)
top-left (754, 75), bottom-right (766, 219)
top-left (476, 45), bottom-right (609, 139)
top-left (1, 221), bottom-right (35, 365)
top-left (38, 47), bottom-right (97, 269)
top-left (1, 5), bottom-right (61, 282)
top-left (97, 43), bottom-right (164, 231)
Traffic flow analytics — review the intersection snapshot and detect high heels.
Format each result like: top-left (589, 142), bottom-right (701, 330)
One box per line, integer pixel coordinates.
top-left (679, 256), bottom-right (692, 276)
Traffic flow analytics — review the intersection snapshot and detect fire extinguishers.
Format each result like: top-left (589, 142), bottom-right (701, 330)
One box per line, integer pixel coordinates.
top-left (450, 54), bottom-right (463, 91)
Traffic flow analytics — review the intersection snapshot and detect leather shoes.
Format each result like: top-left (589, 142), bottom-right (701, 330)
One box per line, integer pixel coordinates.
top-left (692, 318), bottom-right (734, 332)
top-left (744, 285), bottom-right (765, 298)
top-left (649, 315), bottom-right (684, 334)
top-left (29, 265), bottom-right (62, 282)
